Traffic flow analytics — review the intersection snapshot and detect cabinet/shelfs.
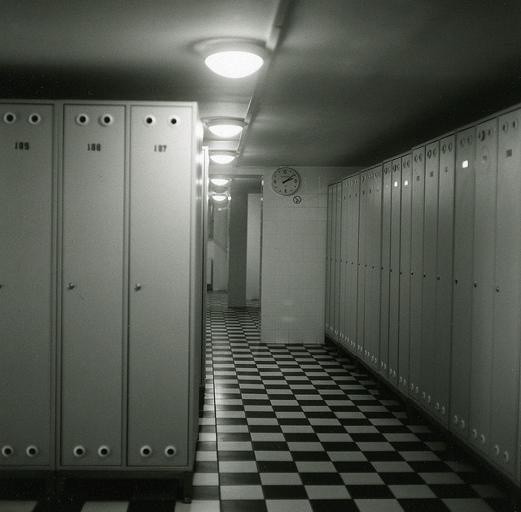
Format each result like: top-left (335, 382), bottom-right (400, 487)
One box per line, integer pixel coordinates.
top-left (62, 95), bottom-right (129, 473)
top-left (325, 100), bottom-right (521, 485)
top-left (1, 96), bottom-right (57, 474)
top-left (126, 97), bottom-right (202, 476)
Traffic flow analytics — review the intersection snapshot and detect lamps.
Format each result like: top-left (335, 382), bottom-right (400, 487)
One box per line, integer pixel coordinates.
top-left (208, 149), bottom-right (239, 166)
top-left (208, 175), bottom-right (233, 202)
top-left (191, 35), bottom-right (272, 79)
top-left (201, 115), bottom-right (249, 138)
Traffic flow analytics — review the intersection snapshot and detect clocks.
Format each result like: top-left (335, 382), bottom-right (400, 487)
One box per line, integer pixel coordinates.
top-left (270, 166), bottom-right (300, 196)
